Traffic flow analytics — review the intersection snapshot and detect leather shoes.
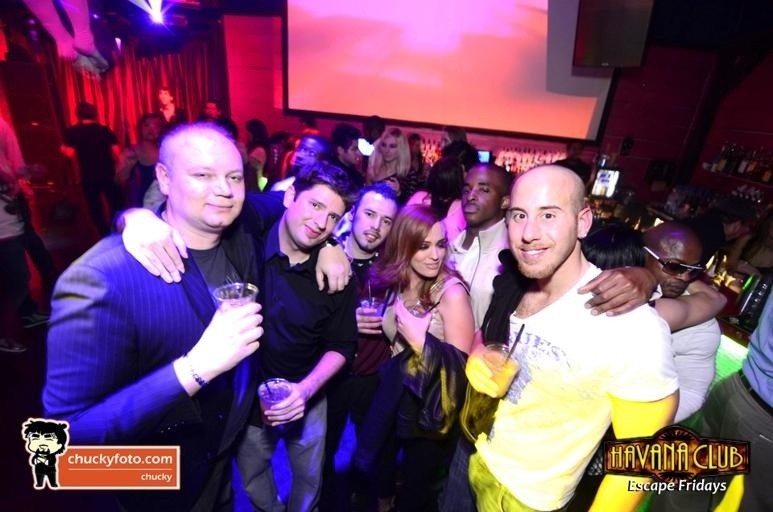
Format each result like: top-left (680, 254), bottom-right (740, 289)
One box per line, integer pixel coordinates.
top-left (375, 495), bottom-right (396, 512)
top-left (347, 492), bottom-right (366, 511)
top-left (416, 408), bottom-right (433, 433)
top-left (390, 469), bottom-right (408, 488)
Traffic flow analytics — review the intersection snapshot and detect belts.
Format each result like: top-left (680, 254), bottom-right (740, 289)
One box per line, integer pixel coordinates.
top-left (737, 370), bottom-right (772, 416)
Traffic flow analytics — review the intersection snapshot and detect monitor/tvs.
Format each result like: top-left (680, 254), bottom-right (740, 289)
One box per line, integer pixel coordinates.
top-left (588, 166), bottom-right (623, 200)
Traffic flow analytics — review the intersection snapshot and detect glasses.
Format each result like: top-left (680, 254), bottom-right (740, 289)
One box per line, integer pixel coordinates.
top-left (643, 245), bottom-right (706, 280)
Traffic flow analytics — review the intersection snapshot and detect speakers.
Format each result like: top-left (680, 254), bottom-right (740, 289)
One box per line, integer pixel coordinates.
top-left (573, 0), bottom-right (655, 68)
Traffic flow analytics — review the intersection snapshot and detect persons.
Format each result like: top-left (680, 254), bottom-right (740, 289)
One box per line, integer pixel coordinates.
top-left (1, 88), bottom-right (772, 512)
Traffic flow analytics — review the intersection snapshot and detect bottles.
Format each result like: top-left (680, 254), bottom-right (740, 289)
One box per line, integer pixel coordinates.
top-left (712, 272), bottom-right (772, 333)
top-left (709, 141), bottom-right (773, 183)
top-left (728, 184), bottom-right (764, 212)
top-left (664, 183), bottom-right (728, 219)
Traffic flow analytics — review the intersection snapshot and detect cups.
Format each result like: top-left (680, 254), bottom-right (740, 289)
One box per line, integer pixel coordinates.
top-left (211, 283), bottom-right (259, 311)
top-left (479, 344), bottom-right (520, 398)
top-left (255, 378), bottom-right (291, 426)
top-left (359, 295), bottom-right (383, 318)
top-left (404, 298), bottom-right (428, 317)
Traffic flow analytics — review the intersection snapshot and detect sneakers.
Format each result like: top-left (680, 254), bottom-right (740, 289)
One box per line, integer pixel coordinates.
top-left (0, 335), bottom-right (28, 354)
top-left (20, 313), bottom-right (49, 328)
